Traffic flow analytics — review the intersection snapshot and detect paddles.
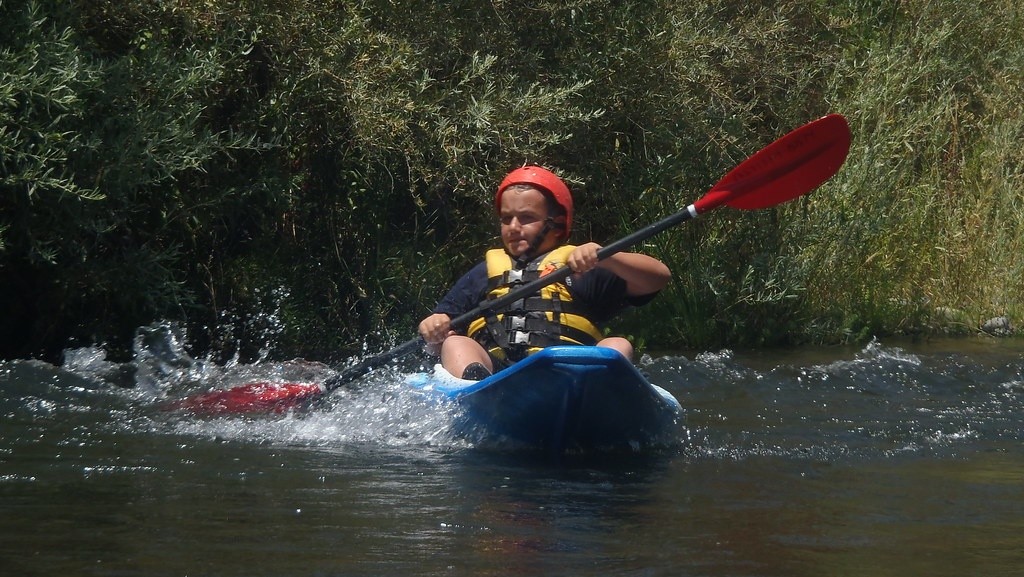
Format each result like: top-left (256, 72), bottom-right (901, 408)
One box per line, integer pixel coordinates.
top-left (185, 112), bottom-right (854, 422)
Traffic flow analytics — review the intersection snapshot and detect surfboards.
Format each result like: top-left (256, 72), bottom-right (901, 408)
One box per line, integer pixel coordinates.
top-left (403, 345), bottom-right (682, 459)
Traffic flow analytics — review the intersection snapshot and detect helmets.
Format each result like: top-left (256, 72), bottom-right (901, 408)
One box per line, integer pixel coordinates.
top-left (496, 167), bottom-right (573, 241)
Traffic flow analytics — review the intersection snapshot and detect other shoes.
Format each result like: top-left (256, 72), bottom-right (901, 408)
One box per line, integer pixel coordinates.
top-left (461, 362), bottom-right (491, 381)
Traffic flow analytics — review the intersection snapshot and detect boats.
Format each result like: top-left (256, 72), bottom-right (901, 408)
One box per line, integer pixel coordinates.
top-left (401, 345), bottom-right (685, 439)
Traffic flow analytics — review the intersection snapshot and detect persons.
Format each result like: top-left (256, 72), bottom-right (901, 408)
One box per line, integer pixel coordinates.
top-left (417, 166), bottom-right (672, 382)
